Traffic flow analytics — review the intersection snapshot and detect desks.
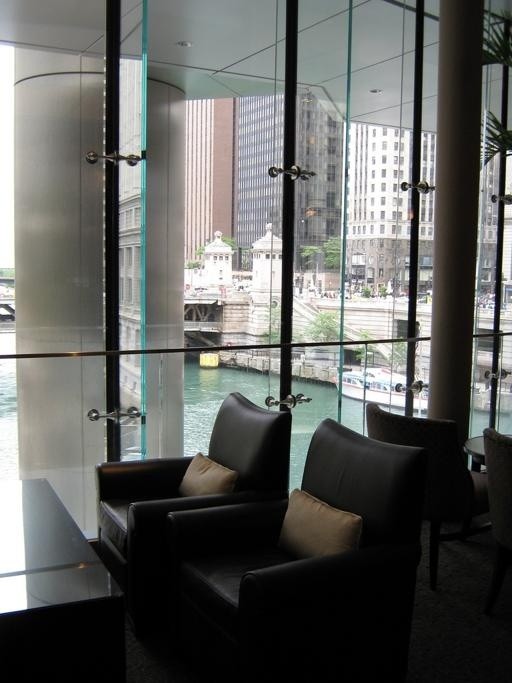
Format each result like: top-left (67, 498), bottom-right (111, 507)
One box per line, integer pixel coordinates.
top-left (0, 478), bottom-right (126, 683)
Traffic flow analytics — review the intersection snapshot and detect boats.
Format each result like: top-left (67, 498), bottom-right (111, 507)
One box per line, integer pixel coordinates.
top-left (333, 371), bottom-right (429, 410)
